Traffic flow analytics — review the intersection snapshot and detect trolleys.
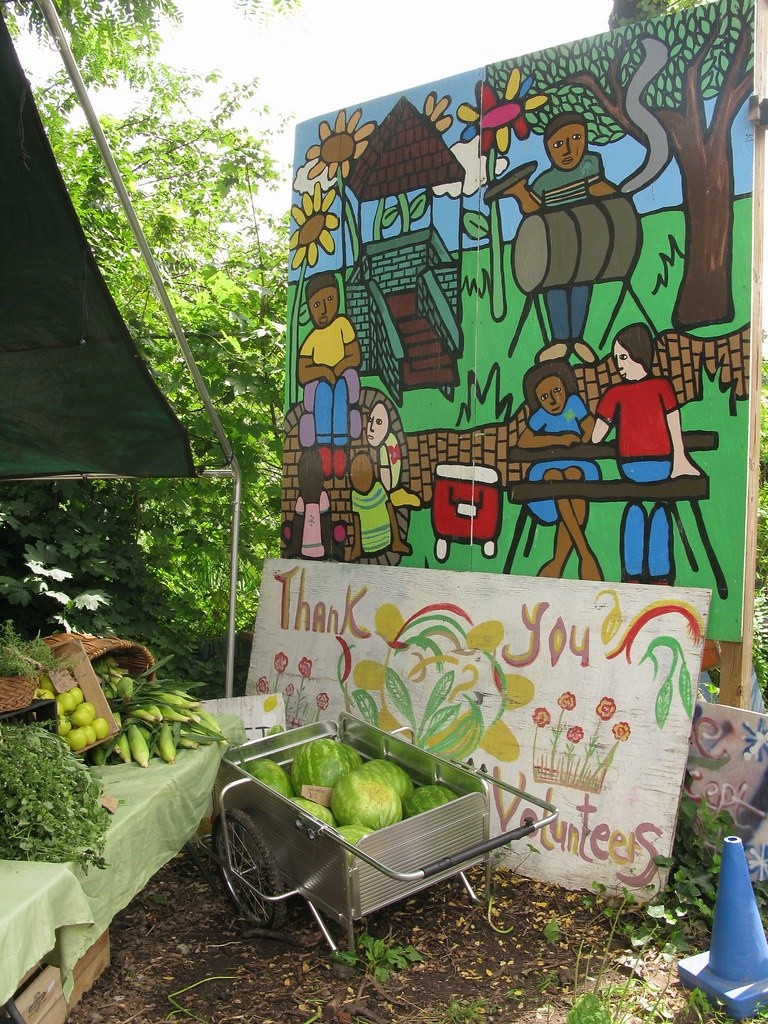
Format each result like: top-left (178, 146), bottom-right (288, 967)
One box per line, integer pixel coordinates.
top-left (184, 709), bottom-right (562, 964)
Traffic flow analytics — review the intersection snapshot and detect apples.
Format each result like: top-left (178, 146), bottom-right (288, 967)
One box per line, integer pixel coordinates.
top-left (33, 676), bottom-right (109, 752)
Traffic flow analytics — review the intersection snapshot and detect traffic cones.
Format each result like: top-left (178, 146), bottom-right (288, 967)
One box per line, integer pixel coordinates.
top-left (677, 834), bottom-right (767, 1022)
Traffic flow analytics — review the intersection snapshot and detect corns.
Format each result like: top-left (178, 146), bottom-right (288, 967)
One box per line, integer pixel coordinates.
top-left (84, 654), bottom-right (230, 767)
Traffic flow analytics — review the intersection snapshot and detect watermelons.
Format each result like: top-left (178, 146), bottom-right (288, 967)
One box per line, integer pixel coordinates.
top-left (242, 738), bottom-right (460, 868)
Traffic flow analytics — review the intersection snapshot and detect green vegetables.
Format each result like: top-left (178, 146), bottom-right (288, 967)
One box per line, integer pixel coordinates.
top-left (0, 617), bottom-right (81, 680)
top-left (0, 715), bottom-right (125, 875)
